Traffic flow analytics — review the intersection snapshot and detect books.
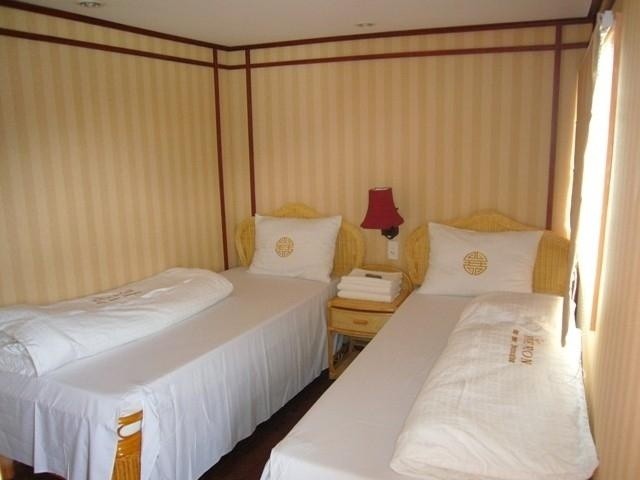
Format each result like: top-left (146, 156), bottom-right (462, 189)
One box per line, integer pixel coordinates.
top-left (336, 268), bottom-right (403, 304)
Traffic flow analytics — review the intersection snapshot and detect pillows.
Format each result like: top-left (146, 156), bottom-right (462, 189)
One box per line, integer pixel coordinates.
top-left (246, 213), bottom-right (342, 283)
top-left (415, 222), bottom-right (543, 296)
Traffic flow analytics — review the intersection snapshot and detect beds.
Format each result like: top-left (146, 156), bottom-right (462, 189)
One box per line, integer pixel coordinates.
top-left (272, 212), bottom-right (576, 480)
top-left (0, 203), bottom-right (363, 480)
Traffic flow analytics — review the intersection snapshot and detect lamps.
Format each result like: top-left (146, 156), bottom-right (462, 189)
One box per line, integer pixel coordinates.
top-left (360, 188), bottom-right (404, 240)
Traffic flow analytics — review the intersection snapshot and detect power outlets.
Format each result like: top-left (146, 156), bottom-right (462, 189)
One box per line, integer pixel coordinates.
top-left (387, 241), bottom-right (398, 260)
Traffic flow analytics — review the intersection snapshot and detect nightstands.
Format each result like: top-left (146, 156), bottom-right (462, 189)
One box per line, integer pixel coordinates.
top-left (327, 284), bottom-right (413, 379)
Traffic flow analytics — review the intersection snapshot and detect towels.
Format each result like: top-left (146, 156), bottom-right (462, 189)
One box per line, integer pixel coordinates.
top-left (337, 268), bottom-right (402, 303)
